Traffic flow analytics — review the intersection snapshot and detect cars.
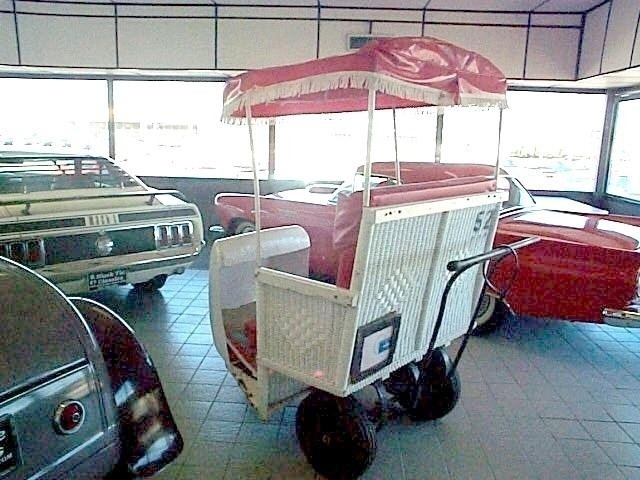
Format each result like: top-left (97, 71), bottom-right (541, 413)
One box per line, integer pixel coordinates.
top-left (0, 257), bottom-right (184, 480)
top-left (212, 155), bottom-right (638, 339)
top-left (1, 152), bottom-right (203, 299)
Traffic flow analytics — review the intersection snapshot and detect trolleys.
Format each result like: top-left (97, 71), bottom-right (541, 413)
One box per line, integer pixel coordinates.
top-left (208, 32), bottom-right (539, 478)
